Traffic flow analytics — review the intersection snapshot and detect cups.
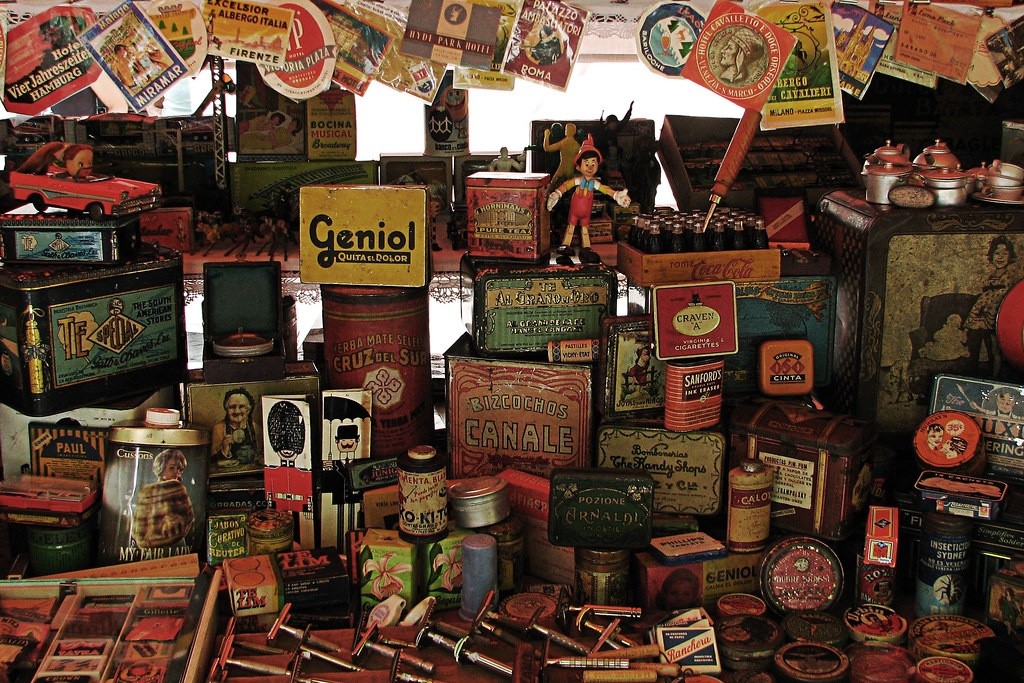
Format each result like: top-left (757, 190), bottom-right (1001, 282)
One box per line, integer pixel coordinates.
top-left (910, 166), bottom-right (969, 209)
top-left (866, 162), bottom-right (909, 205)
top-left (972, 159), bottom-right (1024, 200)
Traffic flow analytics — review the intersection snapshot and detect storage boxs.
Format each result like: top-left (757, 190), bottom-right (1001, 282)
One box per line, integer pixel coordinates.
top-left (0, 117), bottom-right (1024, 683)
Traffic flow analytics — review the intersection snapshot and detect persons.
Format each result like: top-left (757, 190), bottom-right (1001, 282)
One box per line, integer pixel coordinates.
top-left (58, 144), bottom-right (98, 180)
top-left (598, 101), bottom-right (633, 156)
top-left (531, 26), bottom-right (562, 66)
top-left (239, 112), bottom-right (302, 151)
top-left (488, 147), bottom-right (525, 172)
top-left (524, 122), bottom-right (583, 184)
top-left (654, 569), bottom-right (700, 609)
top-left (547, 134), bottom-right (632, 264)
top-left (152, 449), bottom-right (187, 481)
top-left (210, 389), bottom-right (262, 462)
top-left (111, 41), bottom-right (167, 91)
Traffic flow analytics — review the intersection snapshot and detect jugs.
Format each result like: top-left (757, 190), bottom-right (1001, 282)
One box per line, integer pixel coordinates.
top-left (913, 138), bottom-right (961, 170)
top-left (862, 139), bottom-right (910, 171)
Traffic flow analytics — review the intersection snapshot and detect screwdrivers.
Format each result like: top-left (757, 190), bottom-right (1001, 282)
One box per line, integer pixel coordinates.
top-left (702, 106), bottom-right (762, 231)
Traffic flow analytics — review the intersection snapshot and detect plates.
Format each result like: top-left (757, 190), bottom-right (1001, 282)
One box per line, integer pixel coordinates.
top-left (971, 191), bottom-right (1024, 205)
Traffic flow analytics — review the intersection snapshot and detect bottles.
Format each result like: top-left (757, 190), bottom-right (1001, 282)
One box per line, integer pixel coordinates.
top-left (397, 445), bottom-right (449, 545)
top-left (726, 458), bottom-right (773, 553)
top-left (627, 212), bottom-right (769, 255)
top-left (98, 408), bottom-right (212, 567)
